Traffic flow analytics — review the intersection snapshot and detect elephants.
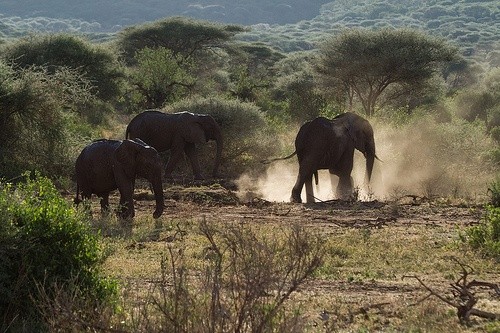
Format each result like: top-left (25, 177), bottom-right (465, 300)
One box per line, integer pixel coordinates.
top-left (266, 112), bottom-right (384, 204)
top-left (73, 137), bottom-right (165, 220)
top-left (125, 109), bottom-right (224, 181)
